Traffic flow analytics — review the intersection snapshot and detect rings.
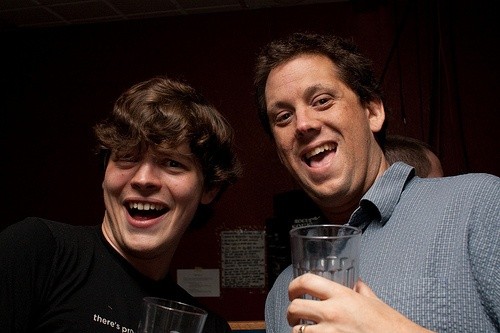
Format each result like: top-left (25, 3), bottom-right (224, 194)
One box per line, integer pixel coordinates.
top-left (298, 323), bottom-right (306, 333)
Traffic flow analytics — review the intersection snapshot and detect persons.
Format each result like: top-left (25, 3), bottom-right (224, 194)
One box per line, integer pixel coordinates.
top-left (253, 31), bottom-right (500, 333)
top-left (376, 137), bottom-right (442, 178)
top-left (0, 77), bottom-right (232, 333)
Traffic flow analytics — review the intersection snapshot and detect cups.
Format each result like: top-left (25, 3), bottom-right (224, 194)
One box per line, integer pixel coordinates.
top-left (290, 225), bottom-right (361, 326)
top-left (138, 297), bottom-right (208, 333)
